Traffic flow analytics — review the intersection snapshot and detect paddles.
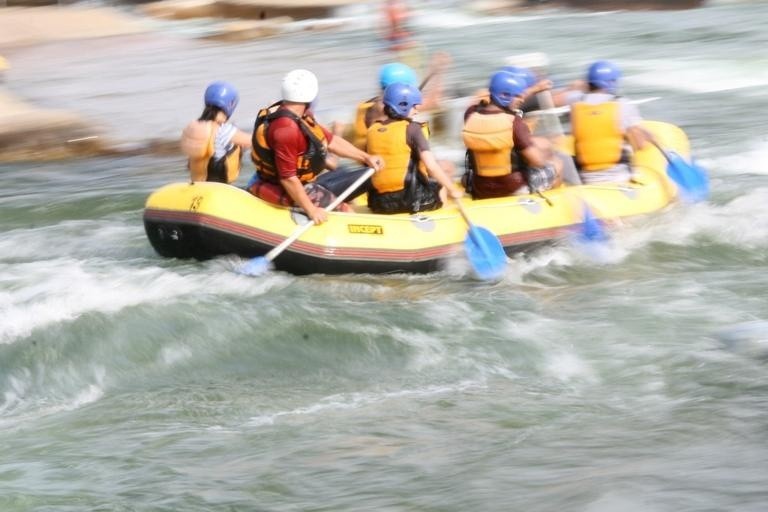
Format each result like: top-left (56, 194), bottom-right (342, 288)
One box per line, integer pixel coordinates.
top-left (536, 89), bottom-right (610, 243)
top-left (238, 167), bottom-right (375, 277)
top-left (454, 199), bottom-right (508, 279)
top-left (645, 133), bottom-right (710, 203)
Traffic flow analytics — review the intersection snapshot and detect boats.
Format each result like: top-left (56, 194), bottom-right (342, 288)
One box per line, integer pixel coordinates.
top-left (142, 121), bottom-right (692, 273)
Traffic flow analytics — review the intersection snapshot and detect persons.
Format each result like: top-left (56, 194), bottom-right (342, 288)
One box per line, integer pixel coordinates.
top-left (499, 62), bottom-right (587, 136)
top-left (362, 81), bottom-right (464, 217)
top-left (180, 80), bottom-right (254, 184)
top-left (568, 57), bottom-right (650, 187)
top-left (353, 46), bottom-right (455, 151)
top-left (244, 67), bottom-right (387, 226)
top-left (460, 70), bottom-right (563, 199)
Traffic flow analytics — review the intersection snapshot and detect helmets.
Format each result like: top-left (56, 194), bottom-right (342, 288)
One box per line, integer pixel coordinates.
top-left (384, 83), bottom-right (423, 118)
top-left (380, 63), bottom-right (414, 90)
top-left (204, 83), bottom-right (237, 118)
top-left (587, 63), bottom-right (622, 89)
top-left (489, 71), bottom-right (527, 108)
top-left (281, 70), bottom-right (319, 104)
top-left (501, 65), bottom-right (536, 87)
